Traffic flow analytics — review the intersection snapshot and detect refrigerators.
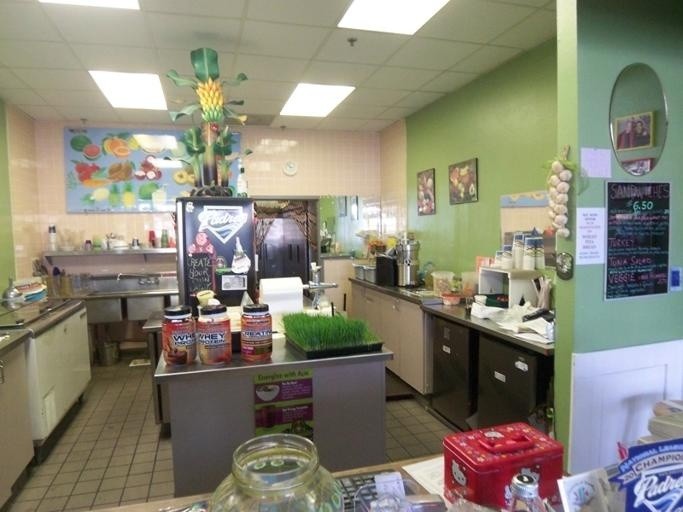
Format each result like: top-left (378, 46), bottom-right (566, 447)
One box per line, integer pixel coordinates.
top-left (173, 196), bottom-right (270, 306)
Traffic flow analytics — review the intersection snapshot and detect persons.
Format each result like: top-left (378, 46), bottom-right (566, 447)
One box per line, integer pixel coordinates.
top-left (617, 120), bottom-right (650, 150)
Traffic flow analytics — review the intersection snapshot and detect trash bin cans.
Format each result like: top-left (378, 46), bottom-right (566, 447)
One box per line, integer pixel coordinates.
top-left (96, 341), bottom-right (120, 367)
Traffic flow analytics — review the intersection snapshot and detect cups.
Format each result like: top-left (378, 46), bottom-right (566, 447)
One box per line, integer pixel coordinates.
top-left (431, 271), bottom-right (455, 298)
top-left (43, 272), bottom-right (90, 297)
top-left (460, 271), bottom-right (477, 295)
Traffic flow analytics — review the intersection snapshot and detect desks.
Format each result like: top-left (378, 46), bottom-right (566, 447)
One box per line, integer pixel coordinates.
top-left (419, 300), bottom-right (554, 437)
top-left (96, 443), bottom-right (566, 512)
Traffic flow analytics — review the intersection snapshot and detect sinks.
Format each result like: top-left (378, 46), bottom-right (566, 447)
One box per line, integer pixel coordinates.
top-left (86, 289), bottom-right (164, 298)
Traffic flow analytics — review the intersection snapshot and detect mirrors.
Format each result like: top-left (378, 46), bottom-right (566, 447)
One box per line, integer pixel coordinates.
top-left (317, 192), bottom-right (376, 260)
top-left (606, 60), bottom-right (672, 179)
top-left (497, 190), bottom-right (558, 269)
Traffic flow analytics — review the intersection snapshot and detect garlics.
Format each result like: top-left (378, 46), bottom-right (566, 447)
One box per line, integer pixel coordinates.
top-left (548, 161), bottom-right (573, 239)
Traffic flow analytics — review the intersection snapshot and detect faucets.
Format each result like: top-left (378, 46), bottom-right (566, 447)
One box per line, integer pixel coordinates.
top-left (116, 272), bottom-right (152, 285)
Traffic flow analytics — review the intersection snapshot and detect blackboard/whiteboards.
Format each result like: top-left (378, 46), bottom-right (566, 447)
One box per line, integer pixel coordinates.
top-left (604, 180), bottom-right (671, 302)
top-left (186, 252), bottom-right (216, 297)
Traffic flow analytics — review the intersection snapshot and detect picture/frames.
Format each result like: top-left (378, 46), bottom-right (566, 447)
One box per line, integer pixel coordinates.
top-left (613, 111), bottom-right (657, 154)
top-left (621, 157), bottom-right (655, 175)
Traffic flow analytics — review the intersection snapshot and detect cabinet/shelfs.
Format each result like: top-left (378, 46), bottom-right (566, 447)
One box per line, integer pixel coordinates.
top-left (349, 280), bottom-right (381, 345)
top-left (378, 288), bottom-right (445, 397)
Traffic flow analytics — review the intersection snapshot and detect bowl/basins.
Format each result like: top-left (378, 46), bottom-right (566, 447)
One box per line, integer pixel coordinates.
top-left (440, 293), bottom-right (460, 305)
top-left (58, 246), bottom-right (75, 252)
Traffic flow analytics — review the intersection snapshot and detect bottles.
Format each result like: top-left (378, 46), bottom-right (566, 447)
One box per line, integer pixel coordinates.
top-left (148, 229), bottom-right (157, 248)
top-left (160, 229), bottom-right (169, 248)
top-left (495, 233), bottom-right (545, 270)
top-left (508, 474), bottom-right (541, 510)
top-left (239, 304), bottom-right (272, 362)
top-left (46, 224), bottom-right (57, 252)
top-left (84, 240), bottom-right (91, 251)
top-left (162, 304), bottom-right (196, 365)
top-left (210, 429), bottom-right (346, 511)
top-left (197, 306), bottom-right (232, 365)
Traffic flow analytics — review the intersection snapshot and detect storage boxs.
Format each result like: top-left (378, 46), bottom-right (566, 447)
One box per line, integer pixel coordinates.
top-left (439, 419), bottom-right (565, 512)
